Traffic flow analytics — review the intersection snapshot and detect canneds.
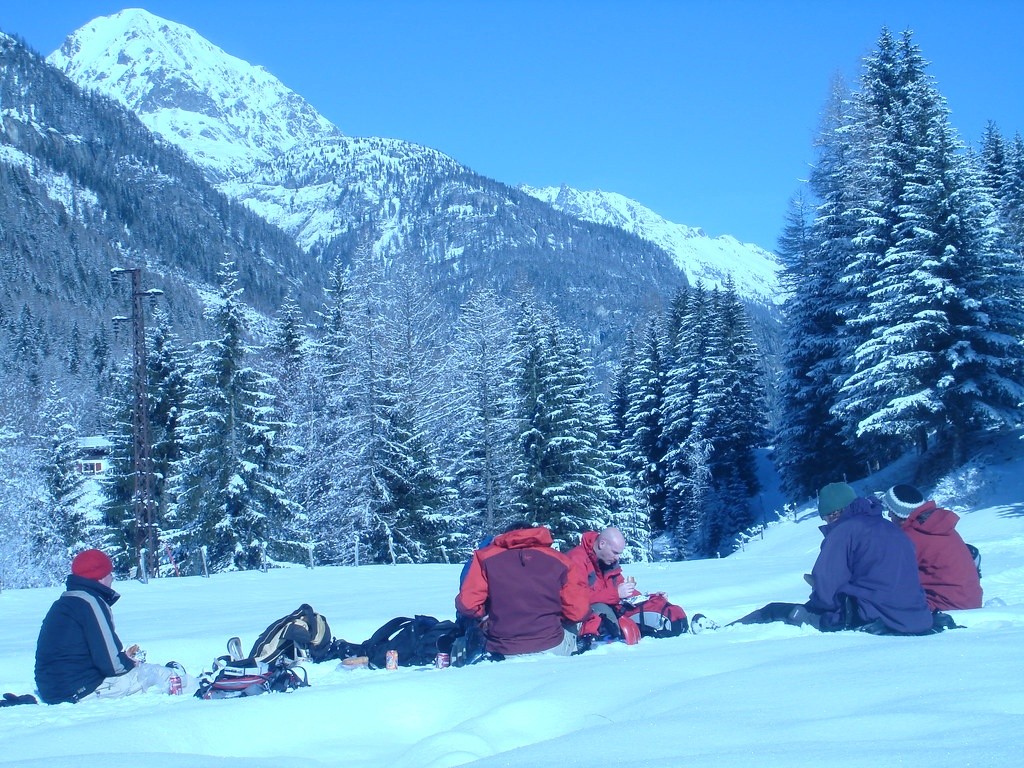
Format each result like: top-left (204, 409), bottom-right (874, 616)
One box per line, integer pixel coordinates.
top-left (386, 650), bottom-right (398, 670)
top-left (435, 652), bottom-right (450, 669)
top-left (168, 676), bottom-right (182, 695)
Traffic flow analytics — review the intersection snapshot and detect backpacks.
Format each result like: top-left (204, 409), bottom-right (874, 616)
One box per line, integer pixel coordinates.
top-left (196, 656), bottom-right (310, 700)
top-left (361, 615), bottom-right (459, 670)
top-left (622, 592), bottom-right (689, 639)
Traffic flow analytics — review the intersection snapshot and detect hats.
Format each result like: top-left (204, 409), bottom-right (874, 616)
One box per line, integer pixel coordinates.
top-left (883, 484), bottom-right (925, 519)
top-left (818, 482), bottom-right (858, 520)
top-left (72, 549), bottom-right (112, 580)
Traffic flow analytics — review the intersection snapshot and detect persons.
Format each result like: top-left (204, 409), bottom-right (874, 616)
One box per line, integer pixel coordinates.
top-left (34, 549), bottom-right (212, 705)
top-left (690, 482), bottom-right (937, 634)
top-left (454, 520), bottom-right (669, 660)
top-left (881, 483), bottom-right (984, 613)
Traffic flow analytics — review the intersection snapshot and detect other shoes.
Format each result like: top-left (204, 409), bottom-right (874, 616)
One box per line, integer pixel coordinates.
top-left (691, 614), bottom-right (718, 634)
